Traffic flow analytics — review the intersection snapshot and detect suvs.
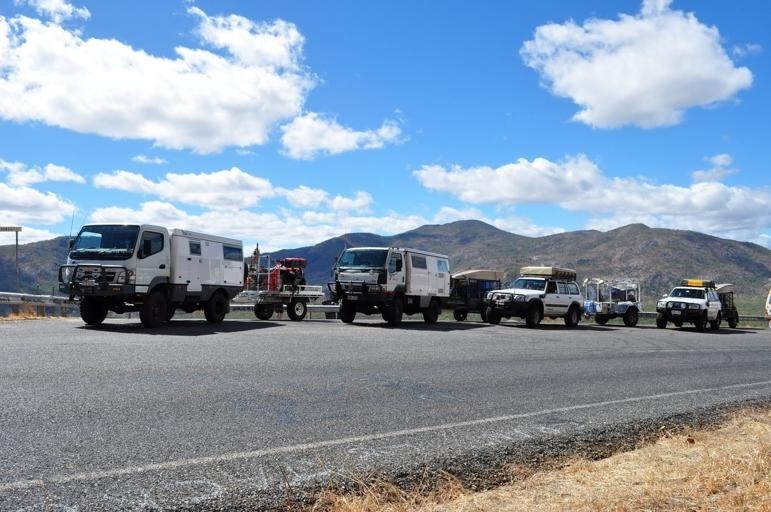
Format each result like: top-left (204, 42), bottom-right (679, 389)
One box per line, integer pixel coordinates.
top-left (656, 278), bottom-right (724, 332)
top-left (485, 265), bottom-right (584, 327)
top-left (713, 281), bottom-right (740, 328)
top-left (446, 268), bottom-right (504, 321)
top-left (584, 276), bottom-right (642, 327)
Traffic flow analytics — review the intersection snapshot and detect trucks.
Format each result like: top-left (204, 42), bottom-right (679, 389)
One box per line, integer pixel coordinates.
top-left (327, 245), bottom-right (452, 325)
top-left (254, 256), bottom-right (325, 320)
top-left (61, 223), bottom-right (251, 333)
